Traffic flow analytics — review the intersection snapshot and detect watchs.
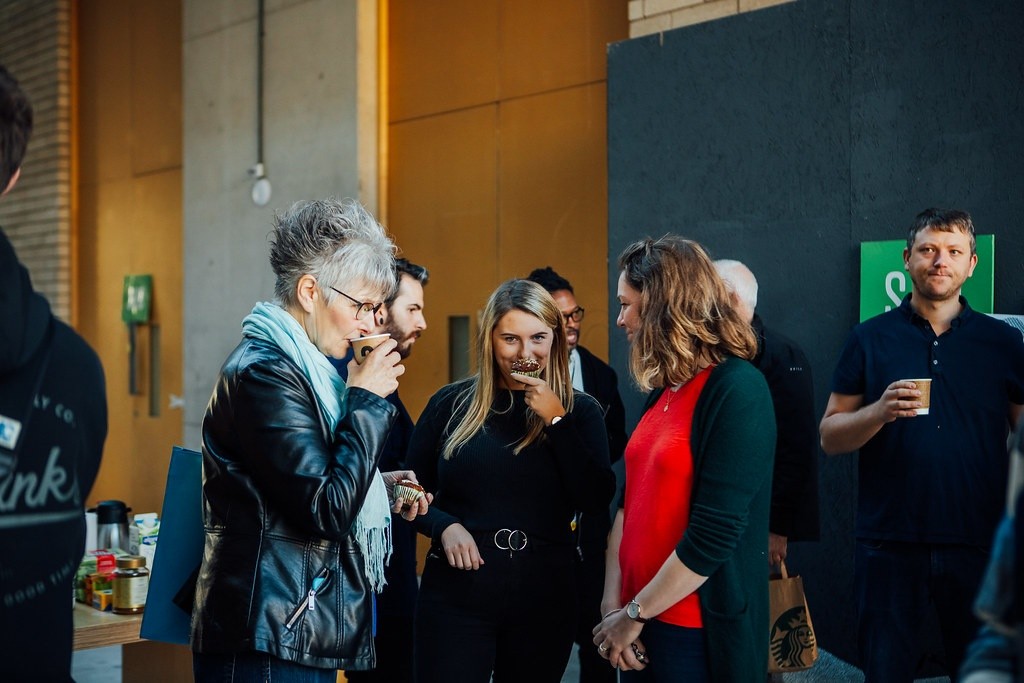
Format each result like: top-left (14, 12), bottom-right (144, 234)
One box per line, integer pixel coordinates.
top-left (550, 415), bottom-right (563, 425)
top-left (626, 597), bottom-right (649, 623)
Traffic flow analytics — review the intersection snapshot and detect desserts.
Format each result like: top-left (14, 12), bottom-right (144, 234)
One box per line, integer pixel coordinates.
top-left (394, 479), bottom-right (427, 507)
top-left (508, 359), bottom-right (539, 384)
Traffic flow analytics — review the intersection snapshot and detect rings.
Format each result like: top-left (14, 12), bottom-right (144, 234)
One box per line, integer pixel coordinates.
top-left (401, 511), bottom-right (405, 516)
top-left (599, 643), bottom-right (606, 652)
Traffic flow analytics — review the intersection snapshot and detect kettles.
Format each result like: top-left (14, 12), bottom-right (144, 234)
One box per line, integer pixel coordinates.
top-left (87, 500), bottom-right (132, 552)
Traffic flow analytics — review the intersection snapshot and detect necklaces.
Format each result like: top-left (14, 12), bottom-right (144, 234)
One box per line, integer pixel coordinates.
top-left (664, 389), bottom-right (676, 412)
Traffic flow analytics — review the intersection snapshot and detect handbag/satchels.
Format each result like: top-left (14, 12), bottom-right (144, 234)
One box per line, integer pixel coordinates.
top-left (140, 447), bottom-right (202, 646)
top-left (768, 558), bottom-right (819, 673)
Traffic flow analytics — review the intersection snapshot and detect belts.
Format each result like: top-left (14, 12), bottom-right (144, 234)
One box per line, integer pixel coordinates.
top-left (472, 528), bottom-right (577, 553)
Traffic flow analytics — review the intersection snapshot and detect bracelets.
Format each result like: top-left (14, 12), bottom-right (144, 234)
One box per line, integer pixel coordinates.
top-left (602, 609), bottom-right (623, 620)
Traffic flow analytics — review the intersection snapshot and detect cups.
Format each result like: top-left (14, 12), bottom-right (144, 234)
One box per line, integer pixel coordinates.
top-left (350, 333), bottom-right (391, 364)
top-left (901, 379), bottom-right (932, 415)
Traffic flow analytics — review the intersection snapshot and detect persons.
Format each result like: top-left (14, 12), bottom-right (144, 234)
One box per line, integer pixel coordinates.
top-left (325, 257), bottom-right (430, 683)
top-left (960, 412), bottom-right (1024, 683)
top-left (529, 265), bottom-right (625, 682)
top-left (401, 279), bottom-right (614, 683)
top-left (191, 196), bottom-right (433, 683)
top-left (818, 208), bottom-right (1024, 683)
top-left (710, 259), bottom-right (819, 566)
top-left (0, 66), bottom-right (108, 683)
top-left (593, 236), bottom-right (776, 683)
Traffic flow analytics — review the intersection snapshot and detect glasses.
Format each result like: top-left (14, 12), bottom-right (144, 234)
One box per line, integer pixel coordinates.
top-left (562, 305), bottom-right (585, 327)
top-left (295, 275), bottom-right (383, 321)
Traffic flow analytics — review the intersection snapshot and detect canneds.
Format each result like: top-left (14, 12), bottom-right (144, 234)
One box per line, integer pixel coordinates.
top-left (111, 555), bottom-right (149, 615)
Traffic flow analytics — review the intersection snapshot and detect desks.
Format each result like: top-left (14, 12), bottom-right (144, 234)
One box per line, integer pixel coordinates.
top-left (73, 601), bottom-right (194, 683)
